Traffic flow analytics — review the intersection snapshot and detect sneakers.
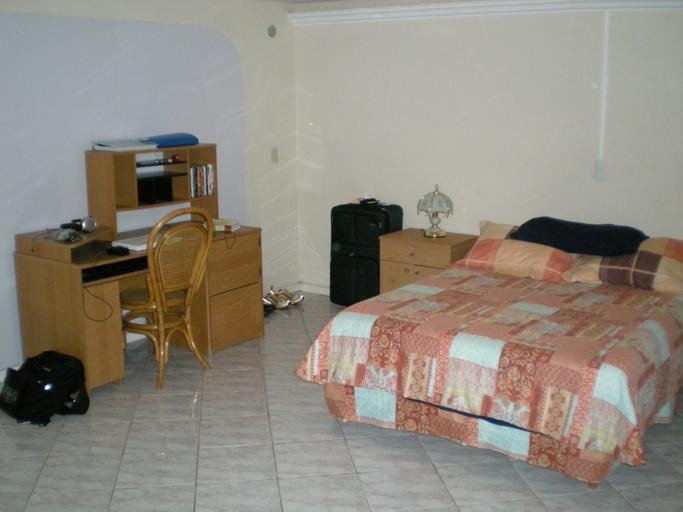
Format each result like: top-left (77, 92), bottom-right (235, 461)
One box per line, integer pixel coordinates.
top-left (263, 288), bottom-right (305, 309)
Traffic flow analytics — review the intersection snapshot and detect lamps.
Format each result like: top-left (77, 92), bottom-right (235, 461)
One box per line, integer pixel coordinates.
top-left (415, 184), bottom-right (454, 237)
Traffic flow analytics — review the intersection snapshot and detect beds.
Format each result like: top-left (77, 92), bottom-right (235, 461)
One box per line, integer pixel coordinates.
top-left (321, 255), bottom-right (683, 486)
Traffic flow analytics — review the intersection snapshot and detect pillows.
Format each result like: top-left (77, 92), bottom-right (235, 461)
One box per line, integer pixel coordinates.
top-left (465, 221), bottom-right (682, 295)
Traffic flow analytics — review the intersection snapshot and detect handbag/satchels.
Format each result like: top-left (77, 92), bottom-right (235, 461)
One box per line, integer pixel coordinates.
top-left (0, 350), bottom-right (90, 426)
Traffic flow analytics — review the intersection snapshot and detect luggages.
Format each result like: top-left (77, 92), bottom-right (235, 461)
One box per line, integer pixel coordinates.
top-left (328, 198), bottom-right (402, 308)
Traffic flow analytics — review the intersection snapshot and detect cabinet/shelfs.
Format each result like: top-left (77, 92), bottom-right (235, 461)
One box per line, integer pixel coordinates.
top-left (151, 232), bottom-right (265, 356)
top-left (85, 143), bottom-right (218, 239)
top-left (83, 282), bottom-right (124, 390)
top-left (378, 228), bottom-right (480, 295)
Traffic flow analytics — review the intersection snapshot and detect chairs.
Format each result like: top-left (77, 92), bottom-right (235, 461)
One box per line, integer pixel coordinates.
top-left (120, 208), bottom-right (214, 391)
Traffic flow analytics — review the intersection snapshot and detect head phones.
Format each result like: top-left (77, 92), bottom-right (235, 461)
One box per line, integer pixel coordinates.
top-left (60, 216), bottom-right (97, 232)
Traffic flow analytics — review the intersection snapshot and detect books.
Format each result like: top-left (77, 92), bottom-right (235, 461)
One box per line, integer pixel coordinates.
top-left (189, 163), bottom-right (214, 199)
top-left (90, 138), bottom-right (158, 154)
top-left (201, 217), bottom-right (242, 232)
top-left (112, 230), bottom-right (180, 252)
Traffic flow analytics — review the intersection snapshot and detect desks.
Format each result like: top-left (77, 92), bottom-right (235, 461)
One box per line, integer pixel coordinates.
top-left (14, 220), bottom-right (265, 397)
